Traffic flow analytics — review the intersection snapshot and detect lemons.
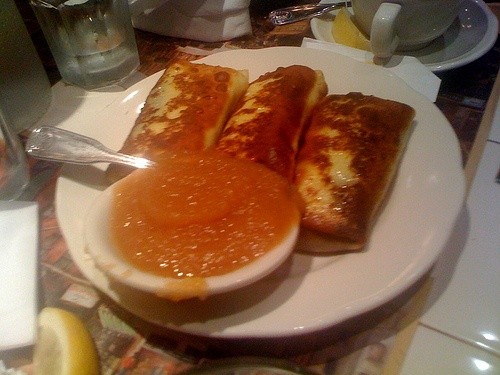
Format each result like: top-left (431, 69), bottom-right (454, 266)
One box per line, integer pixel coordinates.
top-left (332, 7), bottom-right (371, 52)
top-left (33, 307), bottom-right (100, 375)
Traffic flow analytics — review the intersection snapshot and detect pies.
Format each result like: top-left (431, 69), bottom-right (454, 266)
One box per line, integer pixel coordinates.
top-left (101, 61), bottom-right (418, 255)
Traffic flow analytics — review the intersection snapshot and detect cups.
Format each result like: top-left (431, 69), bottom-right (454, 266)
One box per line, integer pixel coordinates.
top-left (30, 0), bottom-right (139, 90)
top-left (350, 0), bottom-right (463, 57)
top-left (0, 98), bottom-right (29, 204)
top-left (0, 0), bottom-right (51, 134)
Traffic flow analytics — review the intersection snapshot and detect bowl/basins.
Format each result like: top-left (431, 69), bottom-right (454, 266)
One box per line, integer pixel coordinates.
top-left (84, 169), bottom-right (300, 298)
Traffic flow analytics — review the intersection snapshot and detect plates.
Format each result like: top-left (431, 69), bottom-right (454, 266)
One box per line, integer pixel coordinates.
top-left (310, 0), bottom-right (498, 73)
top-left (55, 46), bottom-right (464, 339)
